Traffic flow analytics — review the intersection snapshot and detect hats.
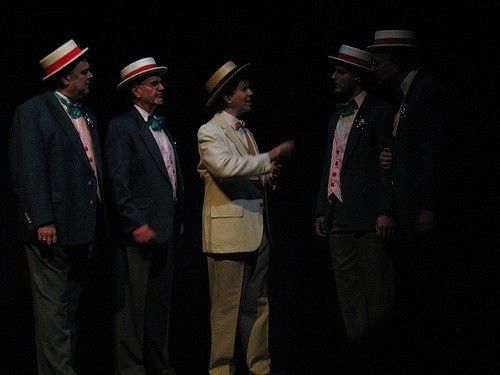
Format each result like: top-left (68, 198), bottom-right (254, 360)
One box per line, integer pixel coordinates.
top-left (368, 29), bottom-right (421, 48)
top-left (205, 61), bottom-right (251, 107)
top-left (38, 38), bottom-right (89, 80)
top-left (116, 57), bottom-right (168, 92)
top-left (328, 44), bottom-right (375, 71)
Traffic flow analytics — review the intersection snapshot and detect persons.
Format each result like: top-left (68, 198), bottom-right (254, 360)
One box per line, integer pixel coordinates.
top-left (368, 30), bottom-right (464, 271)
top-left (108, 57), bottom-right (184, 375)
top-left (197, 61), bottom-right (294, 375)
top-left (315, 44), bottom-right (398, 344)
top-left (14, 39), bottom-right (103, 375)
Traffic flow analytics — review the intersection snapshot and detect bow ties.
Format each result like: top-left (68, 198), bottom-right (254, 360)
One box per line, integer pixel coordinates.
top-left (56, 93), bottom-right (83, 119)
top-left (145, 115), bottom-right (165, 131)
top-left (337, 100), bottom-right (356, 116)
top-left (235, 121), bottom-right (247, 130)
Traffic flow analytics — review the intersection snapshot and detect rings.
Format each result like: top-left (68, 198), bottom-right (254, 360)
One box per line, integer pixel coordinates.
top-left (43, 238), bottom-right (46, 241)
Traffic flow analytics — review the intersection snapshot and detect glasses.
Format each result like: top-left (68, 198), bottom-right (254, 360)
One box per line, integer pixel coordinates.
top-left (135, 80), bottom-right (166, 89)
